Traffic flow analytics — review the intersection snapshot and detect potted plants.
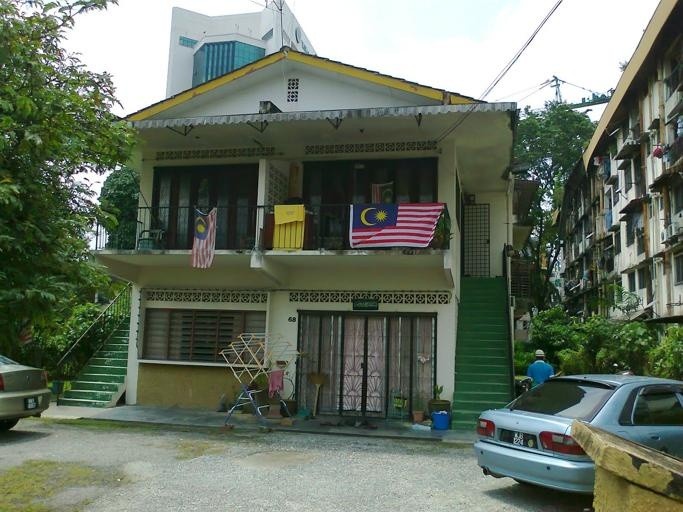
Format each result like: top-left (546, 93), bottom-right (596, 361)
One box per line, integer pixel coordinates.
top-left (429, 384), bottom-right (450, 420)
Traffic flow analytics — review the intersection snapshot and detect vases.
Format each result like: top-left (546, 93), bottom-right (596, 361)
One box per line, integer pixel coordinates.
top-left (280, 399), bottom-right (297, 416)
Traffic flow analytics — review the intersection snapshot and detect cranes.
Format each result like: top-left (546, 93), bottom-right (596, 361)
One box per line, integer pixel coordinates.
top-left (534, 73), bottom-right (615, 112)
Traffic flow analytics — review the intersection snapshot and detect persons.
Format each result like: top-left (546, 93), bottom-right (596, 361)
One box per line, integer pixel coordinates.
top-left (525, 349), bottom-right (560, 393)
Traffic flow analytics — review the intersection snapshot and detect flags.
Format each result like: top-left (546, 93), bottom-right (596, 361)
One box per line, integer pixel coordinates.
top-left (348, 203), bottom-right (443, 250)
top-left (191, 206), bottom-right (218, 271)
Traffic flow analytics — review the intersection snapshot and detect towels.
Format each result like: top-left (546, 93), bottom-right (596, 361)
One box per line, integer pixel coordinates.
top-left (268, 370), bottom-right (282, 398)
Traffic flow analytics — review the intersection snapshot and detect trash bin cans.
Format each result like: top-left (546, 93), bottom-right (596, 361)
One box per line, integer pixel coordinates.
top-left (432, 411), bottom-right (451, 430)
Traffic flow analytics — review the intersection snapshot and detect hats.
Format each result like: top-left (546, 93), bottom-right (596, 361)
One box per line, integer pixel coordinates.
top-left (536, 350), bottom-right (545, 358)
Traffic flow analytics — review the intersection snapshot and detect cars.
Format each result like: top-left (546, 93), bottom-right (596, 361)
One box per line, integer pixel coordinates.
top-left (0, 348), bottom-right (53, 433)
top-left (471, 370), bottom-right (683, 499)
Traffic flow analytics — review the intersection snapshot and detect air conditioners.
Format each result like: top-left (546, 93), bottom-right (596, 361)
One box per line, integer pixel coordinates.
top-left (659, 222), bottom-right (678, 244)
